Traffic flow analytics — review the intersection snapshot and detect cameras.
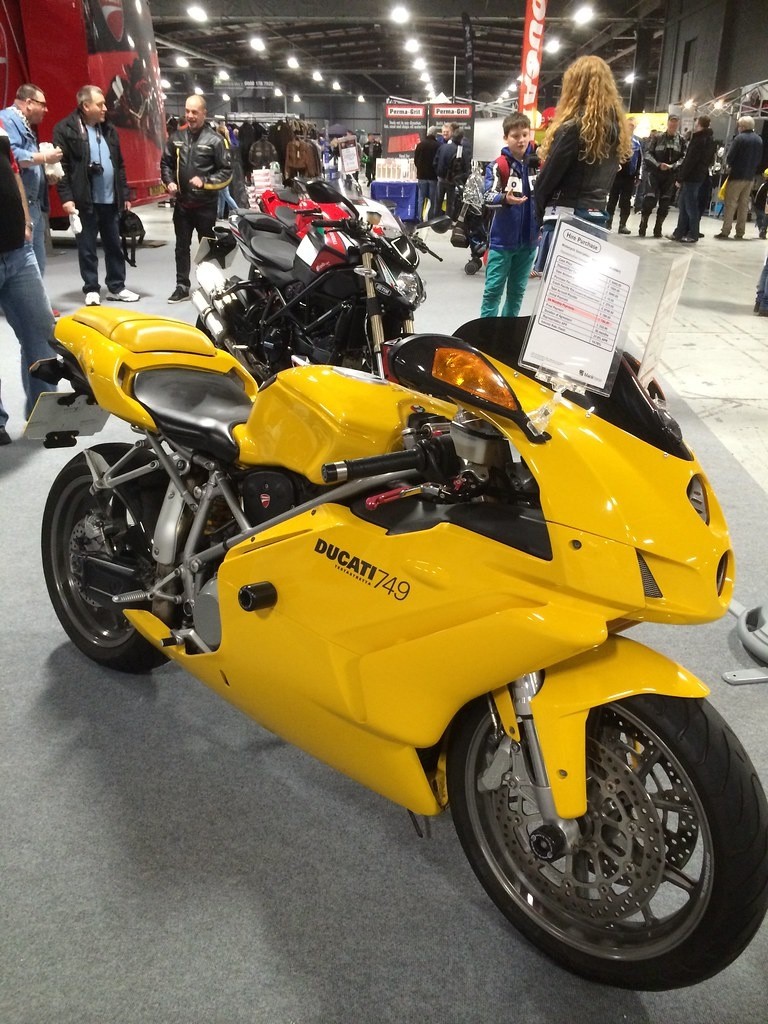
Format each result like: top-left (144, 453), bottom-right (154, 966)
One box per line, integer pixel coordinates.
top-left (88, 161), bottom-right (104, 177)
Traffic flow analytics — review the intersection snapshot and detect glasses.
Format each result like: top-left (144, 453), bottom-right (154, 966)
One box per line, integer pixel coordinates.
top-left (29, 97), bottom-right (46, 107)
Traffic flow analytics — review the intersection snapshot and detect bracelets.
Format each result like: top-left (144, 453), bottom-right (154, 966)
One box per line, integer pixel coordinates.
top-left (24, 220), bottom-right (34, 229)
top-left (43, 154), bottom-right (47, 162)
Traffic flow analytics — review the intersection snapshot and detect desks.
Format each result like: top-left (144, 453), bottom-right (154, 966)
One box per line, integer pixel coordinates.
top-left (370, 182), bottom-right (425, 227)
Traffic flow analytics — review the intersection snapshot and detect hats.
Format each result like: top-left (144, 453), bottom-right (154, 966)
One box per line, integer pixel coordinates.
top-left (669, 114), bottom-right (679, 120)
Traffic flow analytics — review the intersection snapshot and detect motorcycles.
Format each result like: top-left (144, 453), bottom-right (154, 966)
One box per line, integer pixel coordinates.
top-left (188, 175), bottom-right (445, 393)
top-left (20, 213), bottom-right (768, 990)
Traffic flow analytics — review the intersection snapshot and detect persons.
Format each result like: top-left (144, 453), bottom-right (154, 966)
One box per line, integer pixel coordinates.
top-left (363, 133), bottom-right (382, 187)
top-left (605, 116), bottom-right (642, 234)
top-left (534, 55), bottom-right (635, 272)
top-left (715, 116), bottom-right (763, 239)
top-left (434, 129), bottom-right (470, 222)
top-left (666, 114), bottom-right (717, 242)
top-left (225, 127), bottom-right (250, 218)
top-left (437, 123), bottom-right (453, 145)
top-left (414, 125), bottom-right (441, 221)
top-left (0, 83), bottom-right (63, 277)
top-left (0, 128), bottom-right (59, 445)
top-left (753, 257), bottom-right (768, 316)
top-left (640, 114), bottom-right (687, 238)
top-left (345, 130), bottom-right (370, 191)
top-left (451, 123), bottom-right (471, 156)
top-left (217, 126), bottom-right (239, 219)
top-left (480, 112), bottom-right (544, 317)
top-left (160, 95), bottom-right (234, 303)
top-left (752, 168), bottom-right (768, 239)
top-left (54, 85), bottom-right (140, 306)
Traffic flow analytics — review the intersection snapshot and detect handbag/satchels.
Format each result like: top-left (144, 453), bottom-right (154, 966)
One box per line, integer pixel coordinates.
top-left (118, 210), bottom-right (145, 267)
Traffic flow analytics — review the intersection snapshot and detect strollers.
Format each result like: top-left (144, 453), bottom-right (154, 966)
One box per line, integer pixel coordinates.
top-left (450, 167), bottom-right (492, 274)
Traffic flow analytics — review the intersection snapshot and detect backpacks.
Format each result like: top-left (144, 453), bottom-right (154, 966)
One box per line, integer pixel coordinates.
top-left (446, 145), bottom-right (468, 185)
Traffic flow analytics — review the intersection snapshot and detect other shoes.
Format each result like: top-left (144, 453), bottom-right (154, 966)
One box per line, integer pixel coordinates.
top-left (680, 236), bottom-right (697, 242)
top-left (714, 233), bottom-right (728, 237)
top-left (0, 429), bottom-right (11, 445)
top-left (698, 233), bottom-right (704, 237)
top-left (367, 180), bottom-right (372, 187)
top-left (639, 228), bottom-right (645, 237)
top-left (734, 234), bottom-right (743, 239)
top-left (666, 235), bottom-right (677, 241)
top-left (52, 308), bottom-right (60, 317)
top-left (619, 226), bottom-right (631, 234)
top-left (529, 270), bottom-right (543, 278)
top-left (654, 230), bottom-right (663, 237)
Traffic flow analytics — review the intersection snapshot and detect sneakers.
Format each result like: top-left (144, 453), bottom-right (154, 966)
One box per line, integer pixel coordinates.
top-left (168, 286), bottom-right (189, 304)
top-left (85, 291), bottom-right (100, 306)
top-left (106, 287), bottom-right (140, 302)
